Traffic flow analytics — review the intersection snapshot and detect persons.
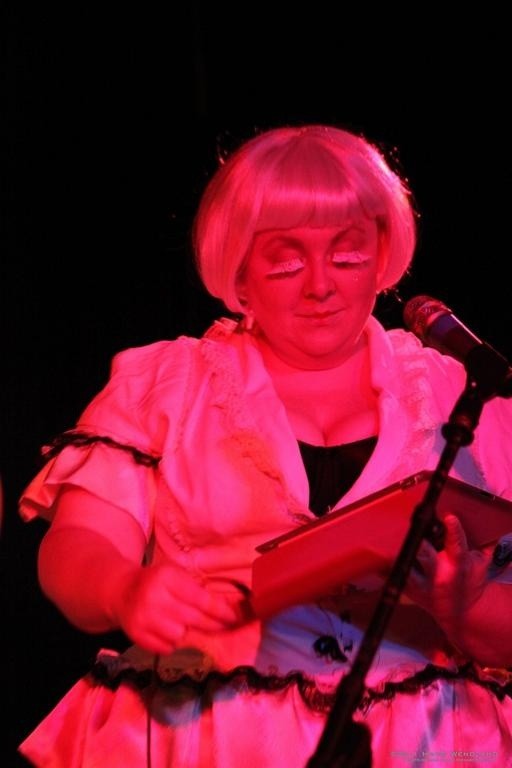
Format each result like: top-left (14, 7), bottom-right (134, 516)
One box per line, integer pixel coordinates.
top-left (11, 120), bottom-right (512, 768)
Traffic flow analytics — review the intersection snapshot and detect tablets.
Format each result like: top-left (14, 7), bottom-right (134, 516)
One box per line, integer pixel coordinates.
top-left (256, 470), bottom-right (512, 556)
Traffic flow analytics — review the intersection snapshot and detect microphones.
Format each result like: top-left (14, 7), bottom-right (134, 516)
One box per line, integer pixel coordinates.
top-left (400, 293), bottom-right (512, 401)
